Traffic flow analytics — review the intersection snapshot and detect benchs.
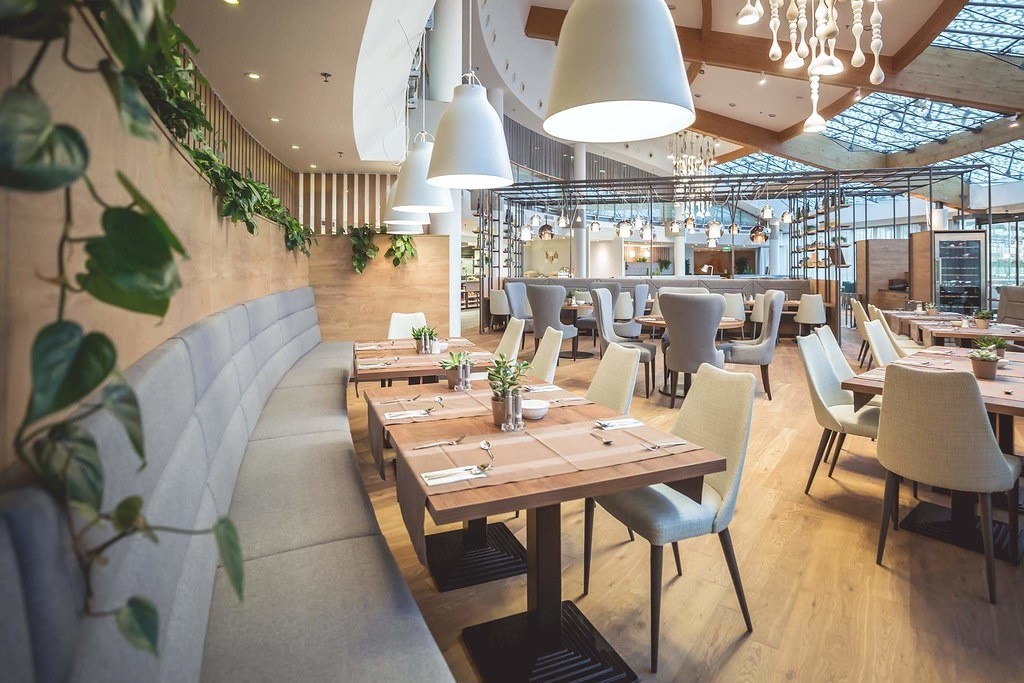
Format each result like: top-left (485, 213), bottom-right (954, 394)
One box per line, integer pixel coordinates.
top-left (1, 287), bottom-right (457, 683)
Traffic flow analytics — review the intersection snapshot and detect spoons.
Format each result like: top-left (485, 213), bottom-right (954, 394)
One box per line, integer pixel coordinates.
top-left (480, 440), bottom-right (496, 461)
top-left (590, 432), bottom-right (613, 444)
top-left (434, 396), bottom-right (445, 409)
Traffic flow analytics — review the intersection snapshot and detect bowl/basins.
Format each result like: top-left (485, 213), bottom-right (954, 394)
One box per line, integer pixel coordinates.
top-left (520, 400), bottom-right (550, 420)
top-left (576, 301), bottom-right (585, 305)
top-left (950, 321), bottom-right (962, 325)
top-left (439, 343), bottom-right (449, 353)
top-left (996, 360), bottom-right (1010, 368)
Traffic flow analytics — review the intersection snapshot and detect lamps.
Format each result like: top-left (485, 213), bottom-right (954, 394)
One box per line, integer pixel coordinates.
top-left (382, 0), bottom-right (464, 236)
top-left (541, 0), bottom-right (696, 144)
top-left (737, 0), bottom-right (885, 134)
top-left (425, 0), bottom-right (515, 190)
top-left (531, 129), bottom-right (794, 247)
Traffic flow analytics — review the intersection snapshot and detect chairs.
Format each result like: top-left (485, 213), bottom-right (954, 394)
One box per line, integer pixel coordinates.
top-left (490, 277), bottom-right (1022, 672)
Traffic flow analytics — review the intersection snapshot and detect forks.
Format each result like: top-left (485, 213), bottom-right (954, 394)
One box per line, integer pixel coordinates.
top-left (413, 433), bottom-right (468, 450)
top-left (640, 442), bottom-right (689, 450)
top-left (381, 394), bottom-right (423, 404)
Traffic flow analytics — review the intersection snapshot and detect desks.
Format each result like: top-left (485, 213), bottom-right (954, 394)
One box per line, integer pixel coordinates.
top-left (347, 297), bottom-right (1023, 646)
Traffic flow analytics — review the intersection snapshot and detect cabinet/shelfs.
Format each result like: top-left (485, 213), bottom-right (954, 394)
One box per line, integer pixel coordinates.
top-left (789, 200), bottom-right (852, 269)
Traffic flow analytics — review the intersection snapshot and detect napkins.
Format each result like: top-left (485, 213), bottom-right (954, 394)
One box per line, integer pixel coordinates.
top-left (384, 409), bottom-right (429, 421)
top-left (528, 385), bottom-right (561, 392)
top-left (595, 417), bottom-right (645, 430)
top-left (421, 464), bottom-right (486, 486)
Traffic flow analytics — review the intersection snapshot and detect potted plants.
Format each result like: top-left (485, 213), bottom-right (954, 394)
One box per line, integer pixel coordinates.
top-left (411, 324), bottom-right (438, 353)
top-left (973, 307), bottom-right (995, 328)
top-left (926, 302), bottom-right (939, 316)
top-left (974, 335), bottom-right (1007, 358)
top-left (441, 351), bottom-right (474, 391)
top-left (967, 350), bottom-right (1001, 380)
top-left (483, 352), bottom-right (535, 427)
